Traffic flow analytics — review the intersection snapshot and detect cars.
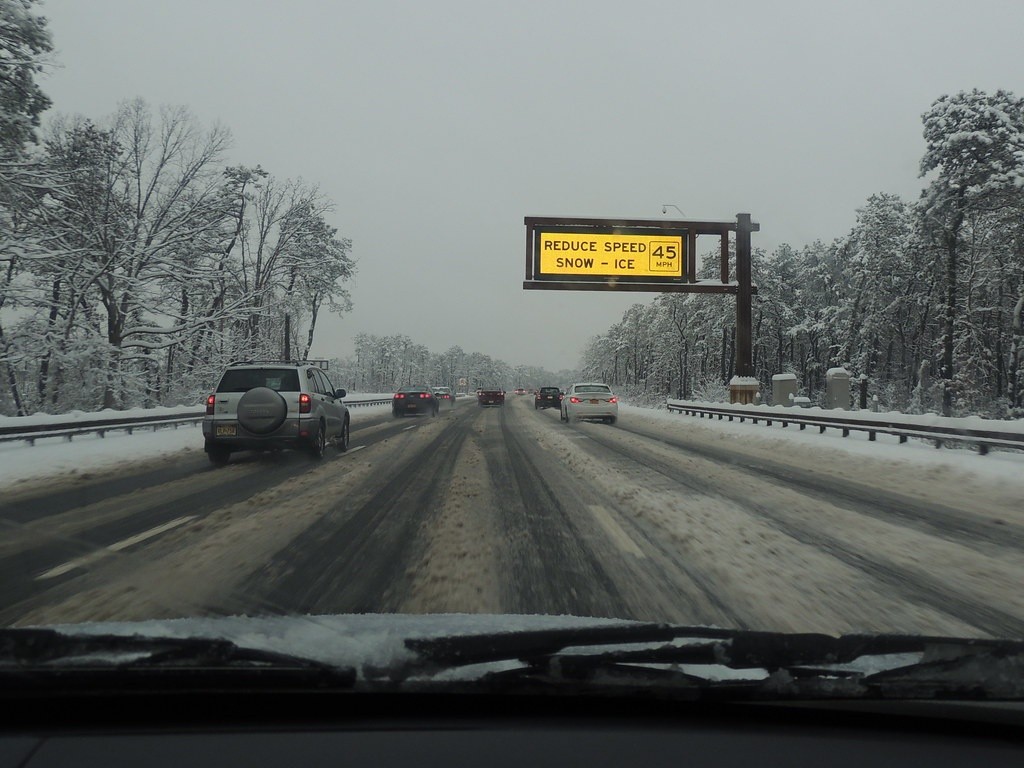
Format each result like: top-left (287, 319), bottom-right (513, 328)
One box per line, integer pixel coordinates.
top-left (392, 384), bottom-right (439, 417)
top-left (432, 387), bottom-right (456, 405)
top-left (534, 387), bottom-right (564, 410)
top-left (559, 383), bottom-right (619, 424)
top-left (515, 388), bottom-right (533, 395)
top-left (477, 387), bottom-right (506, 408)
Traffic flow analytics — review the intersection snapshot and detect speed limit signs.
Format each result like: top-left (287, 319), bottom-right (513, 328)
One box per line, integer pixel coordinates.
top-left (649, 241), bottom-right (679, 272)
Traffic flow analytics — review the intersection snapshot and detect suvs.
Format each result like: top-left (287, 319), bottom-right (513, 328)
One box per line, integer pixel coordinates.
top-left (202, 361), bottom-right (351, 460)
